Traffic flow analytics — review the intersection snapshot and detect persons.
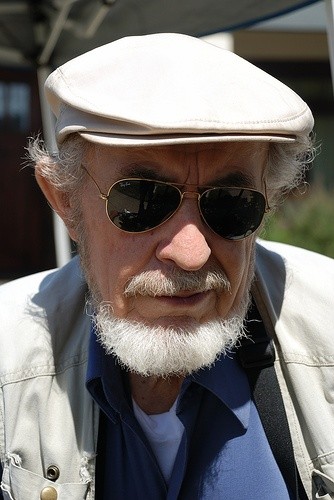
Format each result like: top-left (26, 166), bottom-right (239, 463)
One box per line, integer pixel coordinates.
top-left (0, 33), bottom-right (334, 500)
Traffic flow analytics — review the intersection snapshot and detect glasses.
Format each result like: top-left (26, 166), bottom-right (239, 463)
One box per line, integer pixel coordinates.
top-left (75, 161), bottom-right (272, 242)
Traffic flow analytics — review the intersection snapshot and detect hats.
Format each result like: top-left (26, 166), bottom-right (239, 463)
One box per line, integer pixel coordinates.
top-left (44, 30), bottom-right (315, 148)
